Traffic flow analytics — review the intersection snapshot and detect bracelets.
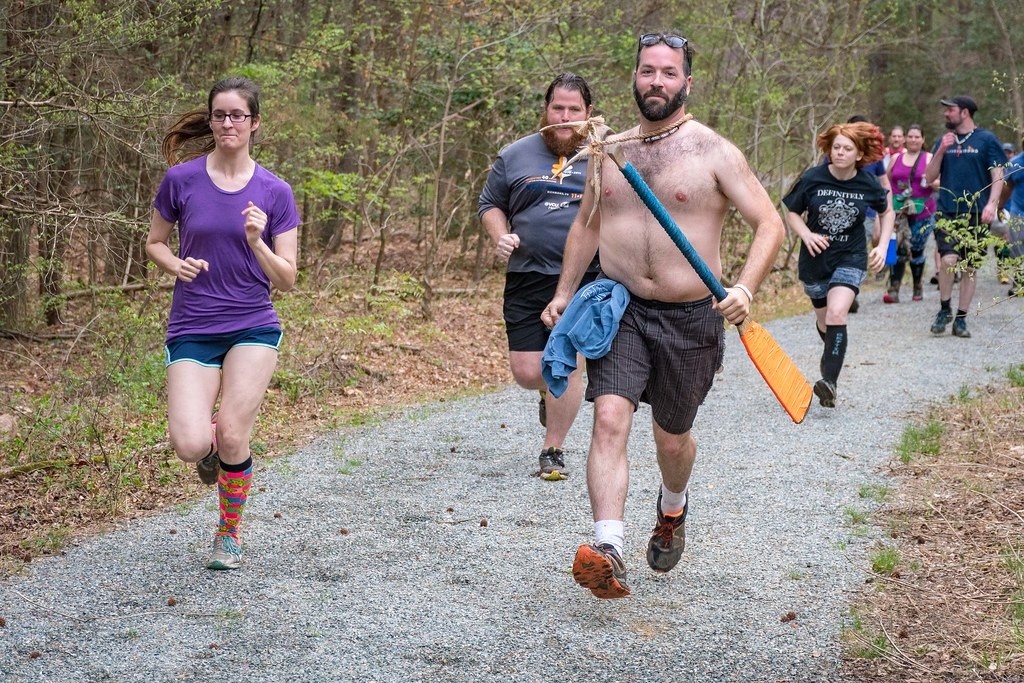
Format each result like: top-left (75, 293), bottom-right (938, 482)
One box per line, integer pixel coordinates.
top-left (732, 284), bottom-right (753, 303)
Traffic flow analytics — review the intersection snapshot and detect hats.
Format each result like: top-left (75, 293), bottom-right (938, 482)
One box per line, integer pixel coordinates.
top-left (1003, 143), bottom-right (1017, 152)
top-left (942, 97), bottom-right (978, 117)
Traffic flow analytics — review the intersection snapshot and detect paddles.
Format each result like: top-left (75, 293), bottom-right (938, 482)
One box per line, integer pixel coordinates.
top-left (607, 142), bottom-right (816, 425)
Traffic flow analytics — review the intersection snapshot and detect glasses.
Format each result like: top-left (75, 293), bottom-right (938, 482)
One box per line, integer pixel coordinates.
top-left (208, 112), bottom-right (254, 123)
top-left (638, 33), bottom-right (689, 49)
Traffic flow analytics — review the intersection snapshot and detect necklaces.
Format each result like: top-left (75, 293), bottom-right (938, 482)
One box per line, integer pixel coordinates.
top-left (638, 123), bottom-right (684, 143)
top-left (954, 130), bottom-right (974, 144)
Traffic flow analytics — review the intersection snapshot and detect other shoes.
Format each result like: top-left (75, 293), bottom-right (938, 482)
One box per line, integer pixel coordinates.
top-left (997, 274), bottom-right (1007, 284)
top-left (1007, 285), bottom-right (1023, 297)
top-left (931, 274), bottom-right (940, 284)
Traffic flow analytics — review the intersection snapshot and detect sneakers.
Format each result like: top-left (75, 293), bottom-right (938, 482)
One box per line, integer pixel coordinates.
top-left (930, 310), bottom-right (953, 332)
top-left (196, 411), bottom-right (220, 485)
top-left (540, 447), bottom-right (570, 480)
top-left (209, 534), bottom-right (243, 569)
top-left (815, 379), bottom-right (837, 408)
top-left (912, 289), bottom-right (924, 301)
top-left (538, 391), bottom-right (547, 429)
top-left (647, 485), bottom-right (690, 573)
top-left (884, 290), bottom-right (899, 302)
top-left (952, 320), bottom-right (970, 337)
top-left (574, 543), bottom-right (631, 597)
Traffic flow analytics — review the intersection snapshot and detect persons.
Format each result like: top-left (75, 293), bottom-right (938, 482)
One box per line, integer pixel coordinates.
top-left (144, 78), bottom-right (300, 569)
top-left (478, 72), bottom-right (603, 481)
top-left (540, 31), bottom-right (785, 596)
top-left (872, 125), bottom-right (1024, 303)
top-left (926, 96), bottom-right (1009, 338)
top-left (823, 114), bottom-right (893, 314)
top-left (781, 123), bottom-right (895, 408)
